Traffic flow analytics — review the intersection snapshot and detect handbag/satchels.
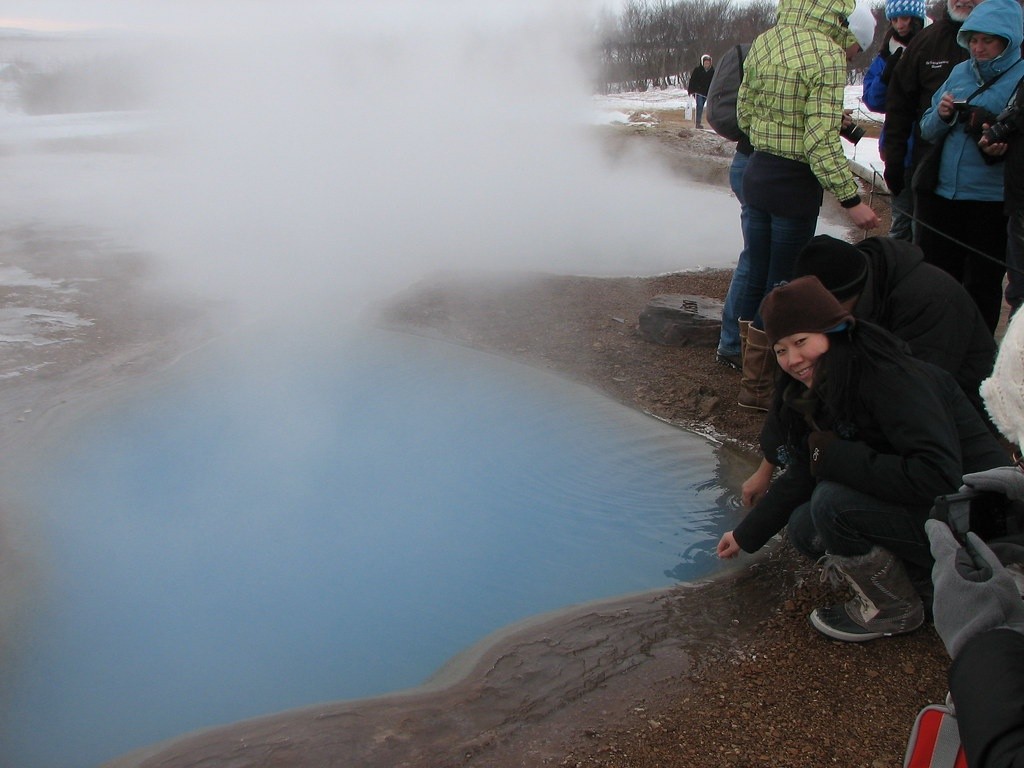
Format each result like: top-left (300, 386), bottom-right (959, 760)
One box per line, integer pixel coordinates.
top-left (903, 704), bottom-right (970, 767)
top-left (909, 140), bottom-right (948, 192)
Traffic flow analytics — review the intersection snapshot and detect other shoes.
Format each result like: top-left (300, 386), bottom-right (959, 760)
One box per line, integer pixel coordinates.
top-left (722, 366), bottom-right (738, 394)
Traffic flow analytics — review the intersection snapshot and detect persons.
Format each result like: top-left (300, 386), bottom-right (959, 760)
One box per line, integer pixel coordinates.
top-left (688, 54), bottom-right (715, 129)
top-left (717, 0), bottom-right (1024, 768)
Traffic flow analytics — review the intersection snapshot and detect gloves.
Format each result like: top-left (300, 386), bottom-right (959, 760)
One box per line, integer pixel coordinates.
top-left (959, 465), bottom-right (1023, 503)
top-left (925, 517), bottom-right (1023, 661)
top-left (881, 46), bottom-right (903, 86)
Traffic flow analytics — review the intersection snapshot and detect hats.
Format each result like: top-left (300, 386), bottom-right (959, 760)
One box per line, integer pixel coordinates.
top-left (794, 234), bottom-right (868, 302)
top-left (886, 0), bottom-right (926, 21)
top-left (759, 272), bottom-right (856, 350)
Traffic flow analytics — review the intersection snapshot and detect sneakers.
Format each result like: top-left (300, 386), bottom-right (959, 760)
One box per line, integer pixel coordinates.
top-left (696, 124), bottom-right (704, 129)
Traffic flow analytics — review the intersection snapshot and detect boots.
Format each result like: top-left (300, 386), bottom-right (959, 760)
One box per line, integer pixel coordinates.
top-left (738, 318), bottom-right (775, 414)
top-left (810, 546), bottom-right (924, 642)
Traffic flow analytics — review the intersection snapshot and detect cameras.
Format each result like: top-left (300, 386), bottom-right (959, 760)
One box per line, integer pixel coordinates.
top-left (935, 489), bottom-right (1008, 544)
top-left (982, 105), bottom-right (1024, 148)
top-left (838, 115), bottom-right (867, 147)
top-left (954, 99), bottom-right (968, 112)
